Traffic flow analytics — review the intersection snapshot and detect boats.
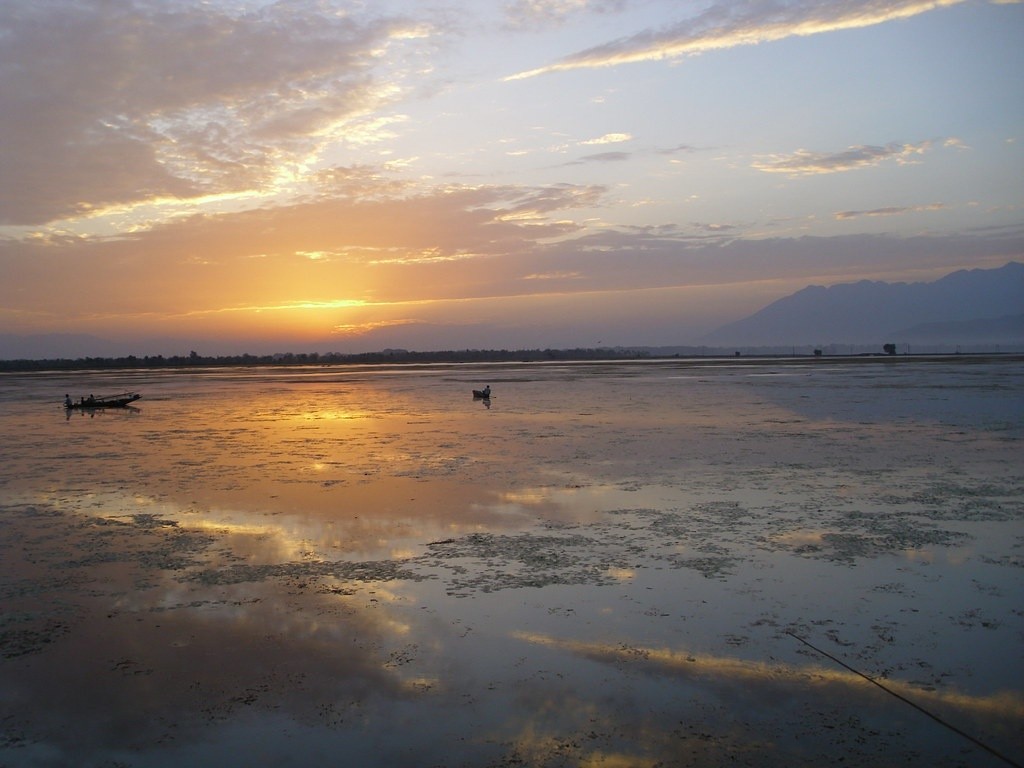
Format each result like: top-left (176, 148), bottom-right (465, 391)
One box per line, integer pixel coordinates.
top-left (70, 394), bottom-right (143, 407)
top-left (472, 390), bottom-right (487, 398)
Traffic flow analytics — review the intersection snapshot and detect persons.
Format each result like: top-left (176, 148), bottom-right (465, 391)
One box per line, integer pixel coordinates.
top-left (482, 385), bottom-right (491, 397)
top-left (88, 393), bottom-right (94, 402)
top-left (63, 394), bottom-right (72, 407)
